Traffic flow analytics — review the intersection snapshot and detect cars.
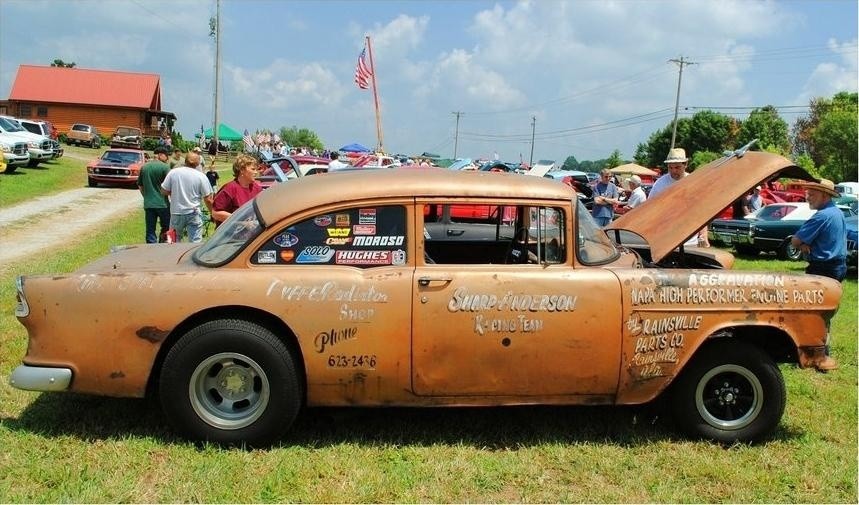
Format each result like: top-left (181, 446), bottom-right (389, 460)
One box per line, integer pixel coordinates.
top-left (704, 197), bottom-right (857, 260)
top-left (9, 147), bottom-right (844, 446)
top-left (283, 163), bottom-right (328, 177)
top-left (0, 111), bottom-right (146, 175)
top-left (833, 183), bottom-right (859, 198)
top-left (587, 173), bottom-right (598, 180)
top-left (86, 147), bottom-right (152, 188)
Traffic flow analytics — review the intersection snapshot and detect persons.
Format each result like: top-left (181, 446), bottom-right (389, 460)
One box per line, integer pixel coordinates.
top-left (273, 140), bottom-right (329, 159)
top-left (623, 175), bottom-right (647, 214)
top-left (136, 135), bottom-right (219, 244)
top-left (401, 156), bottom-right (433, 170)
top-left (327, 152), bottom-right (348, 172)
top-left (732, 197), bottom-right (752, 220)
top-left (212, 154), bottom-right (263, 231)
top-left (748, 185), bottom-right (767, 213)
top-left (791, 179), bottom-right (848, 282)
top-left (592, 168), bottom-right (618, 227)
top-left (647, 148), bottom-right (710, 248)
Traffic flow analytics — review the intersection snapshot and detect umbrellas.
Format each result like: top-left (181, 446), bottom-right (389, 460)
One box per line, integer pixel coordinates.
top-left (339, 142), bottom-right (370, 152)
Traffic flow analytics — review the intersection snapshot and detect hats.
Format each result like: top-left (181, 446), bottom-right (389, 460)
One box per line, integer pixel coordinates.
top-left (625, 175), bottom-right (641, 184)
top-left (193, 147), bottom-right (201, 152)
top-left (155, 147), bottom-right (173, 156)
top-left (802, 179), bottom-right (842, 199)
top-left (663, 147), bottom-right (689, 163)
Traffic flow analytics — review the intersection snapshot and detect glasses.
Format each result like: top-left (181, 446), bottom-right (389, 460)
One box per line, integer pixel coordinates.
top-left (603, 176), bottom-right (611, 178)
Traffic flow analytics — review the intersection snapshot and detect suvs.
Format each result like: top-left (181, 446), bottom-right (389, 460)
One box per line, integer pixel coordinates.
top-left (544, 171), bottom-right (589, 185)
top-left (261, 156), bottom-right (330, 176)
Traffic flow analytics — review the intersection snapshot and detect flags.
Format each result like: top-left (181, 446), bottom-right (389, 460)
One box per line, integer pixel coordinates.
top-left (242, 128), bottom-right (281, 147)
top-left (355, 40), bottom-right (372, 89)
top-left (200, 124), bottom-right (205, 143)
